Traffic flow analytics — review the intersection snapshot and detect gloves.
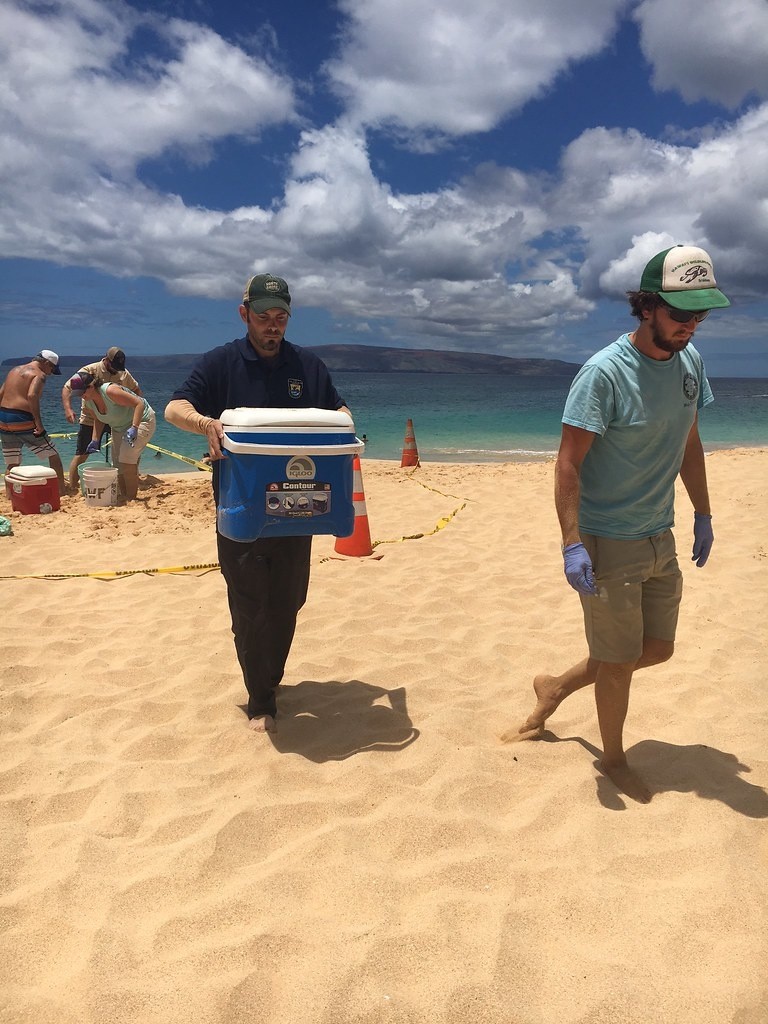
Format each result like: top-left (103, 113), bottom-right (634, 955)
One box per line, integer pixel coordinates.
top-left (691, 511), bottom-right (714, 567)
top-left (84, 440), bottom-right (99, 454)
top-left (125, 425), bottom-right (138, 448)
top-left (562, 542), bottom-right (597, 596)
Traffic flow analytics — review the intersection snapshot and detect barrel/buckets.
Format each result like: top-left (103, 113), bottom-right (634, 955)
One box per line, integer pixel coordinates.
top-left (77, 461), bottom-right (110, 496)
top-left (83, 467), bottom-right (118, 507)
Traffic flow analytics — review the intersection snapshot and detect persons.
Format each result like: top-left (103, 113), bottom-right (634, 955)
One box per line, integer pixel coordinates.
top-left (0, 349), bottom-right (70, 500)
top-left (519, 244), bottom-right (730, 804)
top-left (164, 274), bottom-right (352, 734)
top-left (71, 372), bottom-right (155, 500)
top-left (62, 347), bottom-right (145, 498)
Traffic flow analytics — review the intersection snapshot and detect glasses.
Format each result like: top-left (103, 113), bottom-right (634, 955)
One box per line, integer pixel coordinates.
top-left (658, 303), bottom-right (711, 322)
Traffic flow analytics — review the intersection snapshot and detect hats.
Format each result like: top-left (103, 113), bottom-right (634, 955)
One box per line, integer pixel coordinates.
top-left (640, 244), bottom-right (731, 310)
top-left (40, 349), bottom-right (62, 375)
top-left (242, 272), bottom-right (292, 318)
top-left (70, 372), bottom-right (94, 397)
top-left (106, 346), bottom-right (127, 371)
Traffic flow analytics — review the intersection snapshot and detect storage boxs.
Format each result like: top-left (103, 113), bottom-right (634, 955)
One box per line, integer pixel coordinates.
top-left (217, 406), bottom-right (363, 543)
top-left (5, 464), bottom-right (60, 515)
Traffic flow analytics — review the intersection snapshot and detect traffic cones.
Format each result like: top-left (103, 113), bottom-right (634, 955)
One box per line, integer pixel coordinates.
top-left (334, 455), bottom-right (373, 556)
top-left (399, 418), bottom-right (421, 468)
